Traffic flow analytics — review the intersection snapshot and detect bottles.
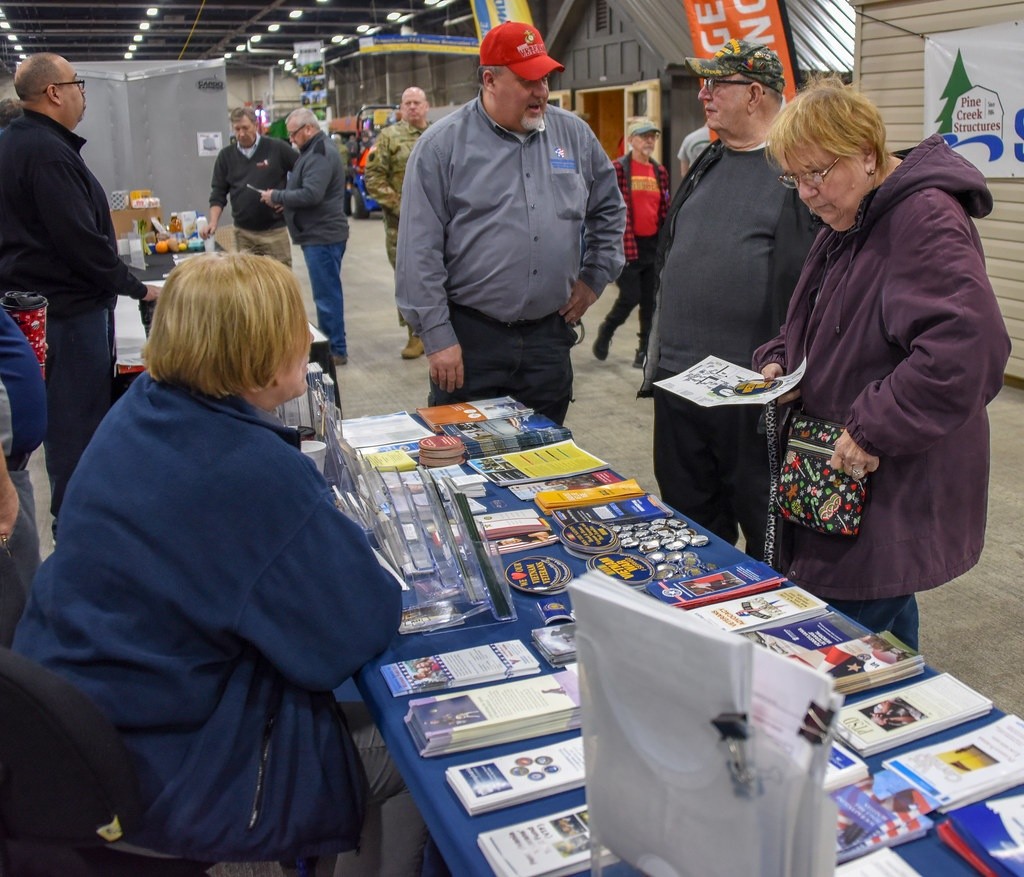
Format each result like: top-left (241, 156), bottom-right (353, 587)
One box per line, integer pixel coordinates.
top-left (169, 212), bottom-right (185, 245)
top-left (196, 213), bottom-right (208, 240)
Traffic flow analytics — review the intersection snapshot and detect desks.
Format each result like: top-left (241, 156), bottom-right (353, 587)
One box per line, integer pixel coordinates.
top-left (330, 413), bottom-right (1024, 877)
top-left (111, 239), bottom-right (341, 418)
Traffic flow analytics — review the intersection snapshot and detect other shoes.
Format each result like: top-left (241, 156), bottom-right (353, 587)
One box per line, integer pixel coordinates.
top-left (332, 353), bottom-right (347, 366)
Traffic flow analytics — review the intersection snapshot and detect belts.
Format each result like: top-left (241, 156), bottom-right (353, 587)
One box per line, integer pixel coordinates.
top-left (5, 453), bottom-right (29, 470)
top-left (449, 301), bottom-right (558, 327)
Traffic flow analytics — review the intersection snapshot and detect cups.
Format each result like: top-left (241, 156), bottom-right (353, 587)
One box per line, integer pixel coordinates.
top-left (0, 290), bottom-right (48, 384)
top-left (301, 440), bottom-right (327, 476)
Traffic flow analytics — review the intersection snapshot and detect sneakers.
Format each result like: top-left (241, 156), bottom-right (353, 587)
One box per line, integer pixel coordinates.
top-left (633, 348), bottom-right (645, 367)
top-left (593, 322), bottom-right (615, 360)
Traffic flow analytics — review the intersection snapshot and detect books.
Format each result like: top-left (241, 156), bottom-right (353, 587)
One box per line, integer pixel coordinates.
top-left (417, 395), bottom-right (575, 460)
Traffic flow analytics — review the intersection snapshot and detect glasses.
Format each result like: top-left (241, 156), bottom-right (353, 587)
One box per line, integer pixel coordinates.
top-left (289, 124), bottom-right (304, 137)
top-left (703, 79), bottom-right (765, 97)
top-left (43, 80), bottom-right (84, 93)
top-left (779, 156), bottom-right (840, 189)
top-left (642, 133), bottom-right (659, 141)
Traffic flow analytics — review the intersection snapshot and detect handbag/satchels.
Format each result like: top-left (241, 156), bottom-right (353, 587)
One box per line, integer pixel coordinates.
top-left (777, 415), bottom-right (868, 536)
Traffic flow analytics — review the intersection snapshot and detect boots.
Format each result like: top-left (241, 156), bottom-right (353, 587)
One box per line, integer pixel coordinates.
top-left (401, 323), bottom-right (426, 359)
top-left (343, 189), bottom-right (352, 216)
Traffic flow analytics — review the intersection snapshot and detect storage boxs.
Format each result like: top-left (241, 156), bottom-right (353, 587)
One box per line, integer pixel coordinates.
top-left (111, 188), bottom-right (161, 241)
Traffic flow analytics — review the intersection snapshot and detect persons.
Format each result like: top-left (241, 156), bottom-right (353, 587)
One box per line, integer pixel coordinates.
top-left (13, 252), bottom-right (427, 875)
top-left (677, 120), bottom-right (710, 178)
top-left (634, 39), bottom-right (820, 557)
top-left (260, 107), bottom-right (349, 364)
top-left (0, 307), bottom-right (48, 647)
top-left (363, 87), bottom-right (430, 359)
top-left (0, 52), bottom-right (160, 545)
top-left (0, 98), bottom-right (23, 128)
top-left (870, 700), bottom-right (919, 731)
top-left (753, 82), bottom-right (1013, 649)
top-left (200, 107), bottom-right (297, 267)
top-left (394, 21), bottom-right (628, 423)
top-left (591, 118), bottom-right (669, 368)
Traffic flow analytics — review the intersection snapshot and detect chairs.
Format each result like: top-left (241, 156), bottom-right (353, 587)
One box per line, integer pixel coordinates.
top-left (0, 647), bottom-right (223, 877)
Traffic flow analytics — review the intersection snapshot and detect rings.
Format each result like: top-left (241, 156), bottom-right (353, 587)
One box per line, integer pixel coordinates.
top-left (853, 468), bottom-right (861, 474)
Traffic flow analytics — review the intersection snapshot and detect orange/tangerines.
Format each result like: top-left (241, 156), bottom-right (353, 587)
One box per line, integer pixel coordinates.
top-left (155, 239), bottom-right (169, 253)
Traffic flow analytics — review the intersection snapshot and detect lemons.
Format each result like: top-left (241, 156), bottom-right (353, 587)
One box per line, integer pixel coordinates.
top-left (179, 243), bottom-right (188, 250)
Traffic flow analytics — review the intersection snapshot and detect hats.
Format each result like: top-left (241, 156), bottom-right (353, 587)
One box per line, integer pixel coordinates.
top-left (626, 116), bottom-right (661, 141)
top-left (480, 21), bottom-right (565, 81)
top-left (684, 39), bottom-right (784, 93)
